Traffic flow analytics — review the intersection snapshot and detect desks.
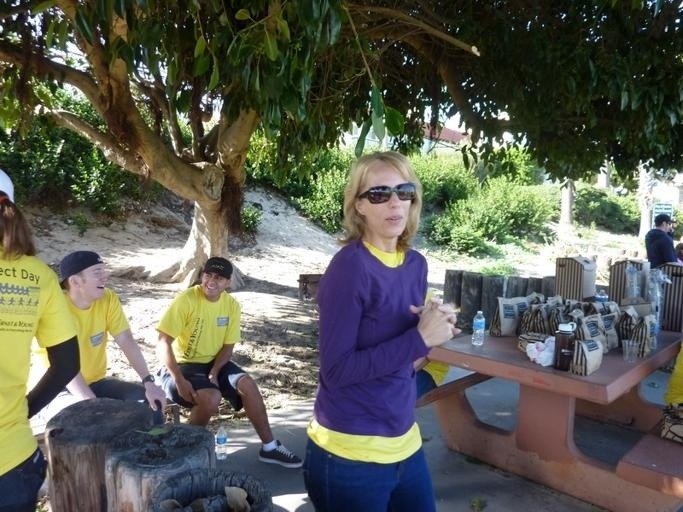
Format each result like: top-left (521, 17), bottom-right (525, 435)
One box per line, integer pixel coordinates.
top-left (426, 320), bottom-right (682, 496)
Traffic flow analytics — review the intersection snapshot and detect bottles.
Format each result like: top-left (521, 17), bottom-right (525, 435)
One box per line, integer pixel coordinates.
top-left (596, 289), bottom-right (608, 304)
top-left (215, 426), bottom-right (228, 461)
top-left (647, 315), bottom-right (657, 350)
top-left (554, 321), bottom-right (577, 370)
top-left (471, 310), bottom-right (486, 346)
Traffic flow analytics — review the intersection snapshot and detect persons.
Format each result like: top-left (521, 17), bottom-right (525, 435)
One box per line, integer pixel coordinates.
top-left (665, 339), bottom-right (683, 416)
top-left (303, 151), bottom-right (462, 512)
top-left (645, 214), bottom-right (678, 269)
top-left (155, 257), bottom-right (304, 468)
top-left (667, 243), bottom-right (683, 266)
top-left (417, 287), bottom-right (450, 399)
top-left (37, 251), bottom-right (168, 426)
top-left (0, 169), bottom-right (80, 512)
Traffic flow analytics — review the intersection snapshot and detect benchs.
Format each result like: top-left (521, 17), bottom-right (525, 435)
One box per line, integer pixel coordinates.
top-left (610, 417), bottom-right (682, 511)
top-left (408, 359), bottom-right (494, 457)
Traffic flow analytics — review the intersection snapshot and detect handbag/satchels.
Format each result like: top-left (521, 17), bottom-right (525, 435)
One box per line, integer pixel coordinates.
top-left (489, 292), bottom-right (657, 376)
top-left (660, 403), bottom-right (683, 442)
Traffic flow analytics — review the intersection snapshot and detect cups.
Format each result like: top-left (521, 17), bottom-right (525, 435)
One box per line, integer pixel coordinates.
top-left (621, 339), bottom-right (641, 363)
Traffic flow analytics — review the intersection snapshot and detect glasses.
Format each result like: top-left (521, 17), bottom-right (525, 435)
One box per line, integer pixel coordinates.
top-left (359, 184), bottom-right (416, 203)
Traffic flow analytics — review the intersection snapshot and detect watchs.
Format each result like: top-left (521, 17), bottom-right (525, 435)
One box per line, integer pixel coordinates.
top-left (142, 375), bottom-right (155, 384)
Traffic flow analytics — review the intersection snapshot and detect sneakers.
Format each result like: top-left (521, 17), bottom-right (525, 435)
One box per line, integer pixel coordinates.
top-left (259, 440), bottom-right (304, 468)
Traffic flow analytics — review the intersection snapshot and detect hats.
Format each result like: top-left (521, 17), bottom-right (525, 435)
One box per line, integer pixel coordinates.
top-left (655, 214), bottom-right (675, 223)
top-left (205, 257), bottom-right (232, 276)
top-left (0, 169), bottom-right (14, 203)
top-left (60, 251), bottom-right (104, 289)
